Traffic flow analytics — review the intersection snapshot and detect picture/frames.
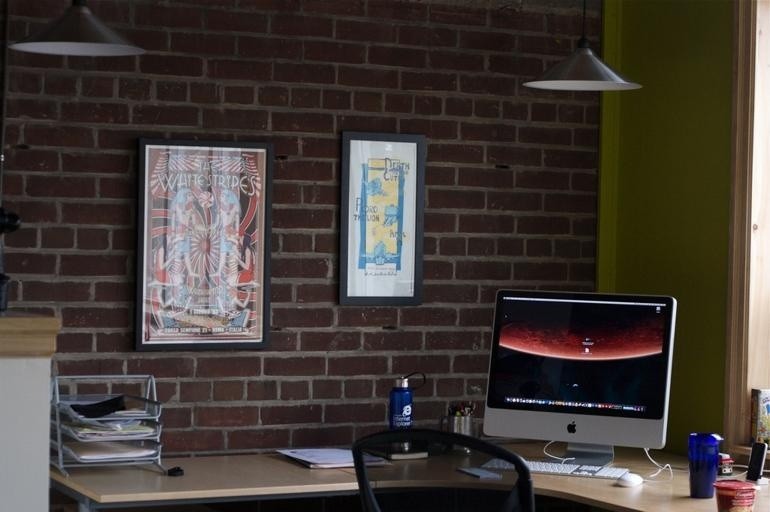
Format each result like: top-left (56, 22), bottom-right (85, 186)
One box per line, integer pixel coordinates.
top-left (335, 132), bottom-right (426, 310)
top-left (137, 139), bottom-right (273, 349)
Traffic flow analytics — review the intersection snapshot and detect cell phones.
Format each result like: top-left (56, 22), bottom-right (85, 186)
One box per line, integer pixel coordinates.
top-left (746, 442), bottom-right (768, 481)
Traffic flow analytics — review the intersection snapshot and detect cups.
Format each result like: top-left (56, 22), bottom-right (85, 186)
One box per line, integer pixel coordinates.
top-left (451, 416), bottom-right (474, 437)
top-left (687, 433), bottom-right (760, 511)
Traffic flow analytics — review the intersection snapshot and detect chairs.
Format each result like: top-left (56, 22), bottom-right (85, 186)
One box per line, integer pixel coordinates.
top-left (353, 427), bottom-right (535, 510)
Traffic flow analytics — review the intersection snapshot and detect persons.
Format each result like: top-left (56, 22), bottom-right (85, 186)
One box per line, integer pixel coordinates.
top-left (150, 186), bottom-right (258, 332)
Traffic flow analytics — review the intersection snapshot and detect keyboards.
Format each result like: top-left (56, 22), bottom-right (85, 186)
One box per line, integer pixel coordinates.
top-left (483, 457), bottom-right (630, 480)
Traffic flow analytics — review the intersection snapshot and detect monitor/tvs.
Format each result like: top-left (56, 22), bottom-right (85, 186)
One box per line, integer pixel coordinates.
top-left (482, 286), bottom-right (678, 471)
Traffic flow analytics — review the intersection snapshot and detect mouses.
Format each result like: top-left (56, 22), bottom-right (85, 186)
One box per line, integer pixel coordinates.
top-left (618, 472), bottom-right (643, 489)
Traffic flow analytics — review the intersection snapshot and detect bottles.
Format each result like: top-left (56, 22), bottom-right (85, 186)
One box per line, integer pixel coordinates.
top-left (388, 372), bottom-right (426, 433)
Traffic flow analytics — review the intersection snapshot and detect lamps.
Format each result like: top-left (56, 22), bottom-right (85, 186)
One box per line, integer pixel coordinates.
top-left (521, 0), bottom-right (643, 93)
top-left (3, 2), bottom-right (150, 58)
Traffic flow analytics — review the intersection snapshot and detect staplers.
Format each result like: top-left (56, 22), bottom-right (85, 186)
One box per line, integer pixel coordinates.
top-left (718, 452), bottom-right (734, 476)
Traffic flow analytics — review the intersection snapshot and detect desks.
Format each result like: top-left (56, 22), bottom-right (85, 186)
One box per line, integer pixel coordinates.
top-left (57, 441), bottom-right (769, 510)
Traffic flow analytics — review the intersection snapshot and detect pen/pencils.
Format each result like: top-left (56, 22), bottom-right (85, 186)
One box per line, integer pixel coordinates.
top-left (448, 401), bottom-right (478, 417)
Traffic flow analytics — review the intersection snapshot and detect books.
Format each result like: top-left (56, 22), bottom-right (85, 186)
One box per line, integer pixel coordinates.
top-left (48, 403), bottom-right (161, 463)
top-left (360, 443), bottom-right (429, 460)
top-left (283, 447), bottom-right (394, 469)
